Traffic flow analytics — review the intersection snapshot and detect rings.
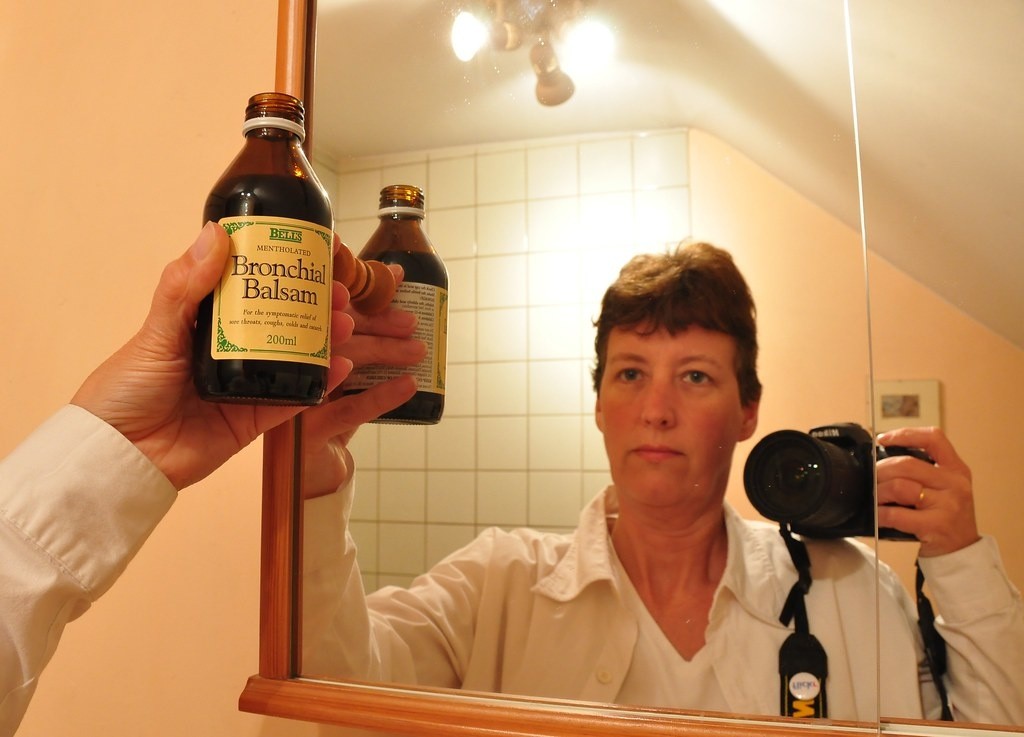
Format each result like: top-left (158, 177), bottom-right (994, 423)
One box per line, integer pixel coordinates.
top-left (914, 486), bottom-right (926, 509)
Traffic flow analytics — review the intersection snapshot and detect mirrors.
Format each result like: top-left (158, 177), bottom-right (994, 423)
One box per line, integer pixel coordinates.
top-left (236, 0), bottom-right (1024, 737)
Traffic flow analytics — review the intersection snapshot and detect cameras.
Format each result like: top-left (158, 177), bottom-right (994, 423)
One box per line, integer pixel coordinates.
top-left (741, 418), bottom-right (938, 541)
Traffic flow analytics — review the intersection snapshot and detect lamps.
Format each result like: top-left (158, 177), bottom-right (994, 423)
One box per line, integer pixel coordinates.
top-left (449, 0), bottom-right (615, 109)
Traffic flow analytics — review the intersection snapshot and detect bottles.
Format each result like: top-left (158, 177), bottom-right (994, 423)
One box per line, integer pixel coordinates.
top-left (357, 184), bottom-right (449, 426)
top-left (191, 91), bottom-right (334, 406)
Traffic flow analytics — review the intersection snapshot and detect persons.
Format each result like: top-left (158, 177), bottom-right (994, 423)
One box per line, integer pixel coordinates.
top-left (0, 217), bottom-right (355, 737)
top-left (301, 234), bottom-right (1024, 727)
